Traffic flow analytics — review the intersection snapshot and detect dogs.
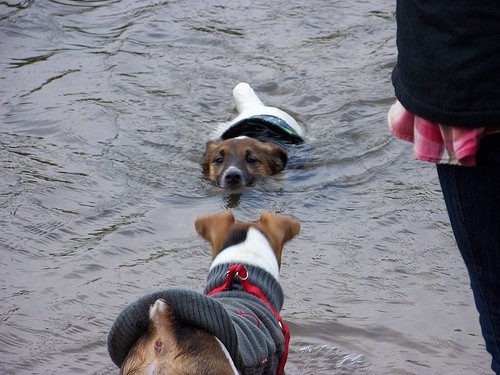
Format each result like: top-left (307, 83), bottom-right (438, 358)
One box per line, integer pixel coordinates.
top-left (199, 81), bottom-right (308, 188)
top-left (120, 212), bottom-right (302, 375)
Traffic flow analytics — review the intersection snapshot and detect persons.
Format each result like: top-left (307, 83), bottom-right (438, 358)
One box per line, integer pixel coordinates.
top-left (387, 0), bottom-right (500, 375)
